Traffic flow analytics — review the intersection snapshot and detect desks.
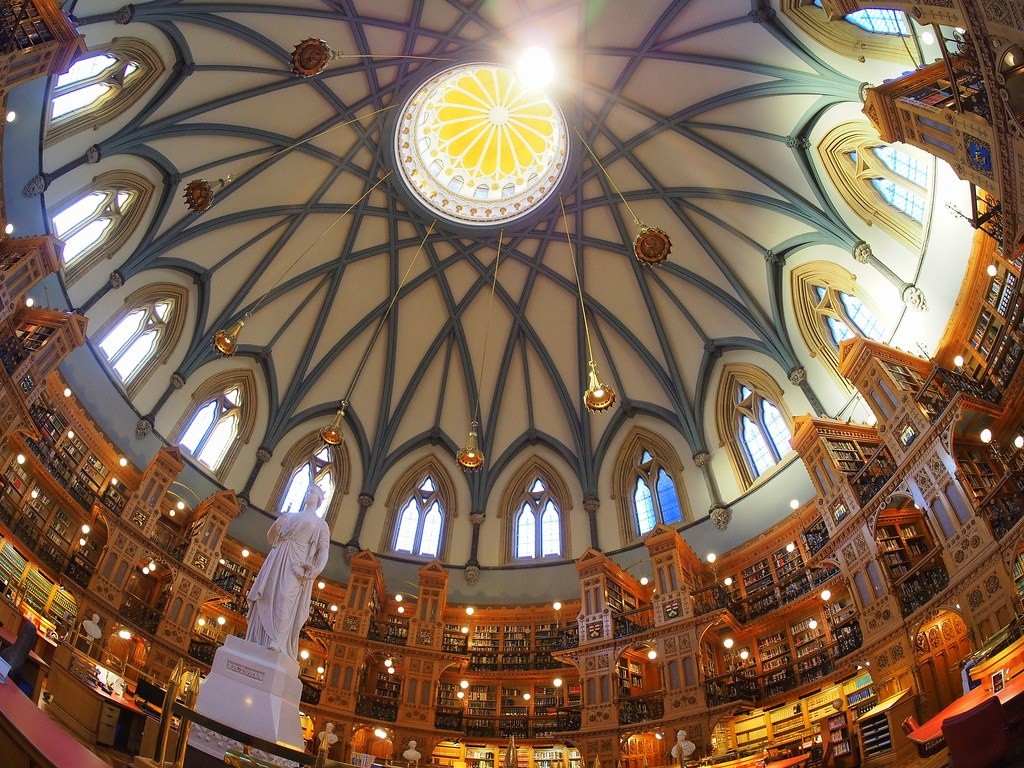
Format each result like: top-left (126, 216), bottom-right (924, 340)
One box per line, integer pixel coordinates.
top-left (906, 637), bottom-right (1023, 746)
top-left (767, 752), bottom-right (811, 768)
top-left (1, 674), bottom-right (111, 768)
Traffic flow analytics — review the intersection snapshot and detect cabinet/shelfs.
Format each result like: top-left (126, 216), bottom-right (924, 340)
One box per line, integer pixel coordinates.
top-left (0, 238), bottom-right (1023, 750)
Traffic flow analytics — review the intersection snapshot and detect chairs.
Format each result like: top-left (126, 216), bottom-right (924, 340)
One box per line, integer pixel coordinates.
top-left (941, 694), bottom-right (1015, 768)
top-left (803, 742), bottom-right (833, 768)
top-left (901, 716), bottom-right (948, 758)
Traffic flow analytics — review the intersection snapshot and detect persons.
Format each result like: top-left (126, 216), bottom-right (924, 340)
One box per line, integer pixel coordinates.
top-left (318, 723), bottom-right (338, 745)
top-left (671, 730), bottom-right (696, 757)
top-left (83, 613), bottom-right (102, 639)
top-left (403, 741), bottom-right (421, 760)
top-left (245, 481), bottom-right (331, 662)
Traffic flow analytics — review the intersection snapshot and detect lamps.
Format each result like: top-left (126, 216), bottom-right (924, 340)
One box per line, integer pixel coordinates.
top-left (183, 35), bottom-right (678, 471)
